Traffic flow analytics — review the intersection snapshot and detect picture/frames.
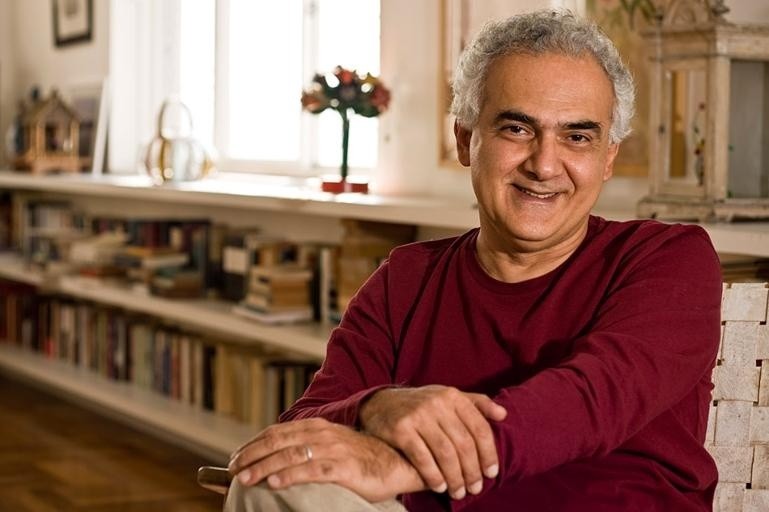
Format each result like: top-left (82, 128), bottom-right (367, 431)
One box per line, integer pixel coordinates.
top-left (51, 0), bottom-right (95, 48)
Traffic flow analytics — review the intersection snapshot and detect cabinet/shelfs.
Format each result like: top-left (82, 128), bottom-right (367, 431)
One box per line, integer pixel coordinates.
top-left (3, 169), bottom-right (767, 469)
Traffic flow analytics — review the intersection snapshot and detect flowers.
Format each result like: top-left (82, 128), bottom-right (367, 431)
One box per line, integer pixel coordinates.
top-left (301, 66), bottom-right (389, 177)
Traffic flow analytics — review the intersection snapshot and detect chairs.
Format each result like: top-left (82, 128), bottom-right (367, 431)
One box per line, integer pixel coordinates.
top-left (197, 283), bottom-right (767, 512)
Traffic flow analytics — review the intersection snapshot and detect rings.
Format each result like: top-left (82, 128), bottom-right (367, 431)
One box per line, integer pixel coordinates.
top-left (304, 444), bottom-right (313, 461)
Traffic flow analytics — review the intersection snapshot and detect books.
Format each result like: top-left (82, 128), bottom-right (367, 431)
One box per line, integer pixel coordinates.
top-left (0, 198), bottom-right (390, 325)
top-left (0, 279), bottom-right (320, 427)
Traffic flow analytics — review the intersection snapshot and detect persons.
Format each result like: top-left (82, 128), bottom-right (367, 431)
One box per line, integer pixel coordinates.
top-left (214, 8), bottom-right (726, 512)
